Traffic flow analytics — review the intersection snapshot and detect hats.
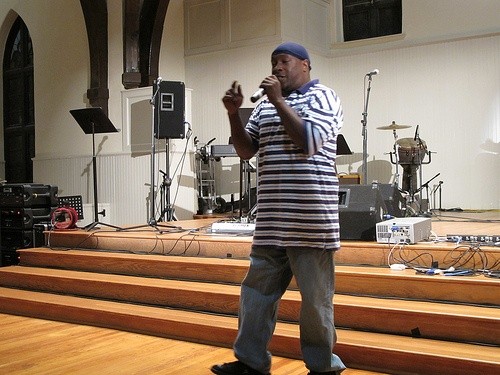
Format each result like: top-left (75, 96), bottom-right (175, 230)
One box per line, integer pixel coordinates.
top-left (271, 42), bottom-right (310, 65)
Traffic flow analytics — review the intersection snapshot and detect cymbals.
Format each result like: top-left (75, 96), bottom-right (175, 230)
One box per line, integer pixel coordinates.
top-left (376, 124), bottom-right (412, 130)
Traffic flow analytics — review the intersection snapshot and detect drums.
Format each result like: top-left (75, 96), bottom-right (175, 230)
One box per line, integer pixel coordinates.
top-left (393, 138), bottom-right (427, 192)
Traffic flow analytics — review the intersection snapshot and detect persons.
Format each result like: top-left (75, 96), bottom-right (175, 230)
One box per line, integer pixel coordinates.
top-left (210, 43), bottom-right (346, 375)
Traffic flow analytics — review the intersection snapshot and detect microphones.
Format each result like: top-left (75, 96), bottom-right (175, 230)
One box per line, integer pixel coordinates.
top-left (157, 76), bottom-right (162, 84)
top-left (367, 69), bottom-right (379, 75)
top-left (413, 125), bottom-right (419, 140)
top-left (250, 86), bottom-right (267, 102)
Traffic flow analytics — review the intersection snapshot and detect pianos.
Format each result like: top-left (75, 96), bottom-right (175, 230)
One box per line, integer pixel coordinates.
top-left (211, 145), bottom-right (258, 158)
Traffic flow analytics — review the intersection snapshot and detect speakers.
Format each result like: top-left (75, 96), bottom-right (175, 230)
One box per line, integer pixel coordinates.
top-left (338, 183), bottom-right (407, 240)
top-left (153, 79), bottom-right (185, 138)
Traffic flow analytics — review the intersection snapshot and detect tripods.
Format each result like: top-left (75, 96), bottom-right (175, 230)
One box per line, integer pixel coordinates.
top-left (116, 98), bottom-right (182, 234)
top-left (68, 106), bottom-right (123, 231)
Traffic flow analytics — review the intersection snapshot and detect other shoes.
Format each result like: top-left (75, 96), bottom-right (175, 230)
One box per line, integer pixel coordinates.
top-left (210, 359), bottom-right (274, 375)
